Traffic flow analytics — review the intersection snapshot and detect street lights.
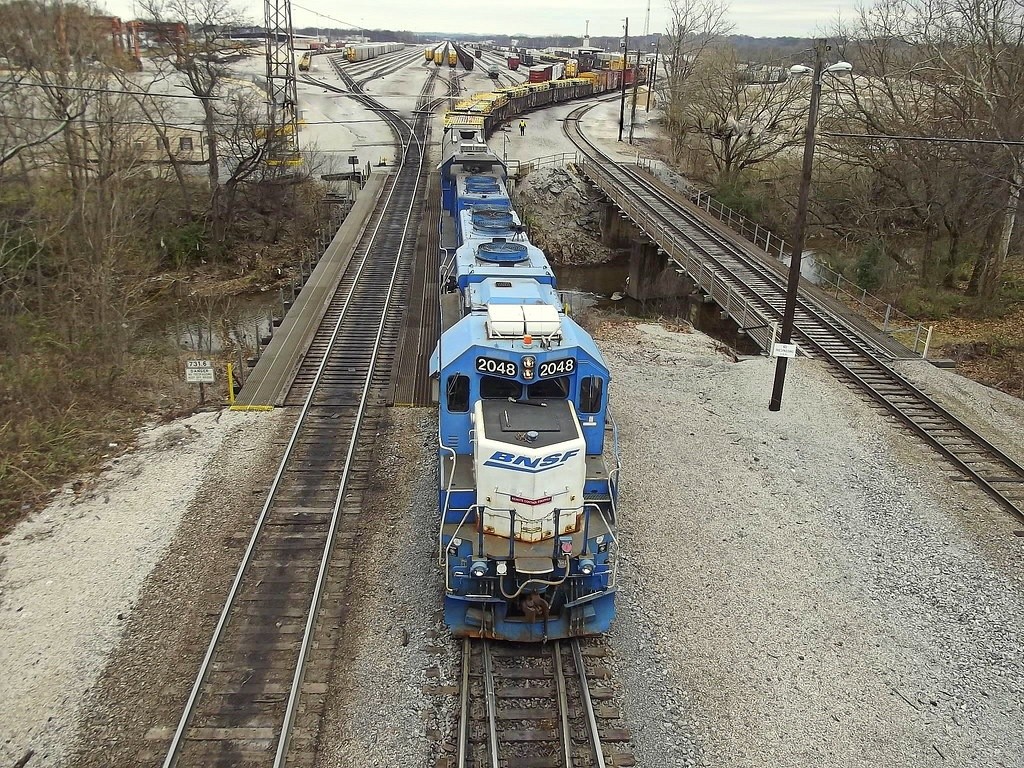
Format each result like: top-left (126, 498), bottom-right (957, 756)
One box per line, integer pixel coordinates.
top-left (768, 39), bottom-right (853, 411)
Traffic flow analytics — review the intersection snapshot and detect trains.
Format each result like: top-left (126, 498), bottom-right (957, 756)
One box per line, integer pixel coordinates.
top-left (428, 61), bottom-right (654, 643)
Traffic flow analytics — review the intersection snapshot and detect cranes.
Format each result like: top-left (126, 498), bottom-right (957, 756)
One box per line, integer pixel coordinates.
top-left (257, 0), bottom-right (305, 179)
